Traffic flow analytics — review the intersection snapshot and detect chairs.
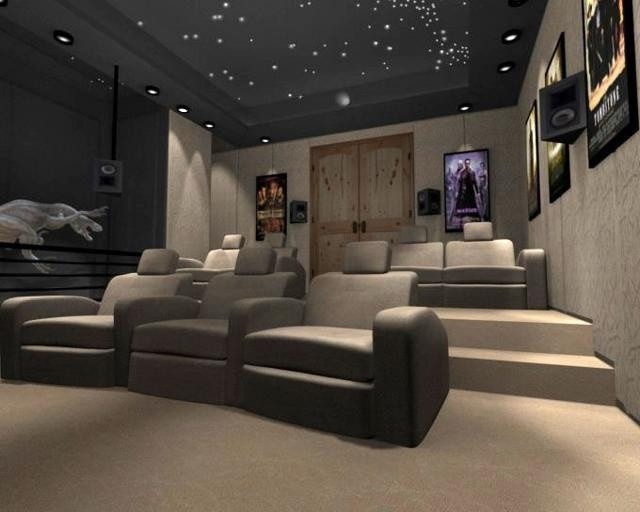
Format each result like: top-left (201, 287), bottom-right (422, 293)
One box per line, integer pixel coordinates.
top-left (1, 248), bottom-right (197, 388)
top-left (234, 242), bottom-right (450, 448)
top-left (117, 247), bottom-right (298, 413)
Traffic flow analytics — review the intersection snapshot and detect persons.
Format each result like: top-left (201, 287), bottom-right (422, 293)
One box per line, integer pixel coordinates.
top-left (257, 180), bottom-right (284, 233)
top-left (448, 158), bottom-right (486, 226)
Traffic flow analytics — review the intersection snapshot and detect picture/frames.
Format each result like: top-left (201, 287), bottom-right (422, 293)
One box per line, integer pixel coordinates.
top-left (253, 173), bottom-right (291, 244)
top-left (440, 147), bottom-right (494, 236)
top-left (522, 0), bottom-right (639, 222)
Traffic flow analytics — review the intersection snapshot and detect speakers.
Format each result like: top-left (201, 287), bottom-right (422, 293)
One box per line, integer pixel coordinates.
top-left (95, 160), bottom-right (123, 194)
top-left (417, 188), bottom-right (441, 216)
top-left (539, 71), bottom-right (587, 144)
top-left (290, 200), bottom-right (307, 223)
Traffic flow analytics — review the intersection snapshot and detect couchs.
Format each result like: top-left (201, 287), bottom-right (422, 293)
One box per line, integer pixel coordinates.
top-left (386, 220), bottom-right (549, 311)
top-left (174, 232), bottom-right (306, 300)
